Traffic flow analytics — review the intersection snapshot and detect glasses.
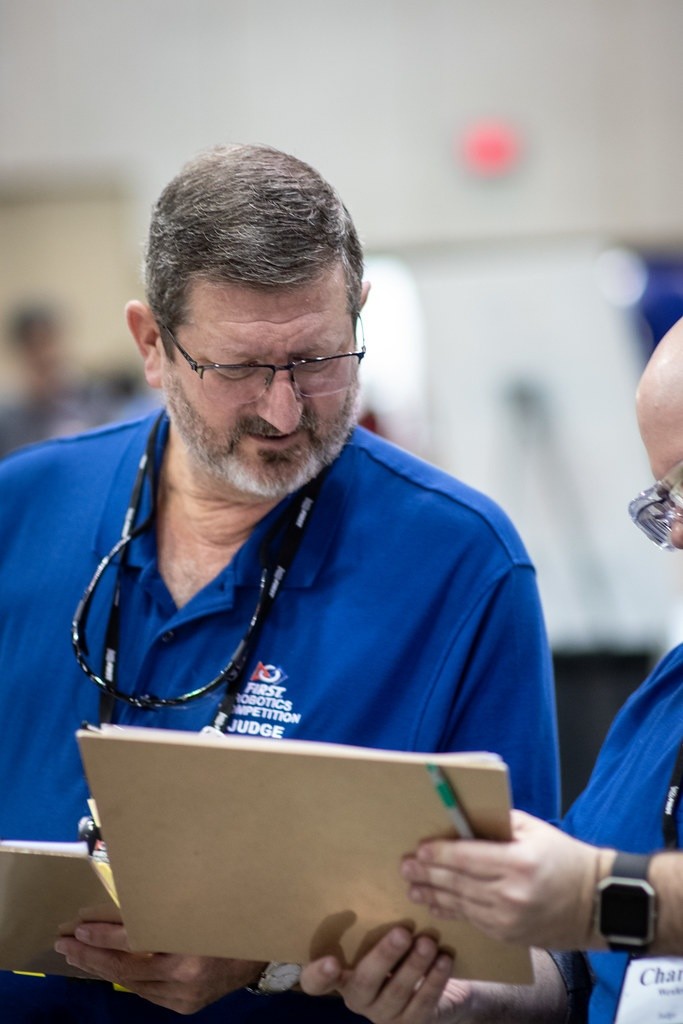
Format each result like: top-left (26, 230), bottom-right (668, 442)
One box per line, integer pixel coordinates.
top-left (627, 462), bottom-right (683, 551)
top-left (162, 311), bottom-right (366, 408)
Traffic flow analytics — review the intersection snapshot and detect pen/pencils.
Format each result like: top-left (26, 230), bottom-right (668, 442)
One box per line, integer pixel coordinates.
top-left (427, 762), bottom-right (476, 841)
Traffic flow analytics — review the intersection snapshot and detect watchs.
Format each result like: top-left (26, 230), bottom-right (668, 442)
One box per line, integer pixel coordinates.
top-left (598, 851), bottom-right (660, 956)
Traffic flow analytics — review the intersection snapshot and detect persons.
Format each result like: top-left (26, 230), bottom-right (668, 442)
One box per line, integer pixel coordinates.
top-left (300, 310), bottom-right (683, 1024)
top-left (0, 307), bottom-right (146, 454)
top-left (0, 148), bottom-right (560, 1024)
